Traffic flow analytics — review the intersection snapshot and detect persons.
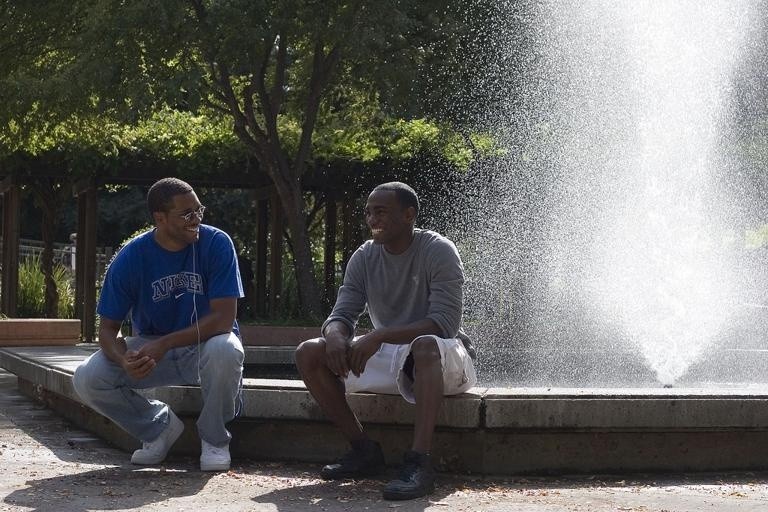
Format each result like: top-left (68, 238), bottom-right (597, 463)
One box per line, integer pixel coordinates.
top-left (71, 176), bottom-right (246, 471)
top-left (294, 179), bottom-right (478, 502)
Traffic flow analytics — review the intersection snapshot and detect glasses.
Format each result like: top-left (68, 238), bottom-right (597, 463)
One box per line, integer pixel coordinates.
top-left (164, 206), bottom-right (206, 220)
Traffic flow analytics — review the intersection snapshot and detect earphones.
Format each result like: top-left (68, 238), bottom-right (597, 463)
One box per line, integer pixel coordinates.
top-left (197, 377), bottom-right (202, 385)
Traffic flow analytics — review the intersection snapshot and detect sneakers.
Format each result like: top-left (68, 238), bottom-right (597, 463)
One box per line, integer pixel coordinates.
top-left (199, 439), bottom-right (231, 472)
top-left (130, 406), bottom-right (184, 465)
top-left (383, 450), bottom-right (435, 500)
top-left (320, 440), bottom-right (387, 479)
